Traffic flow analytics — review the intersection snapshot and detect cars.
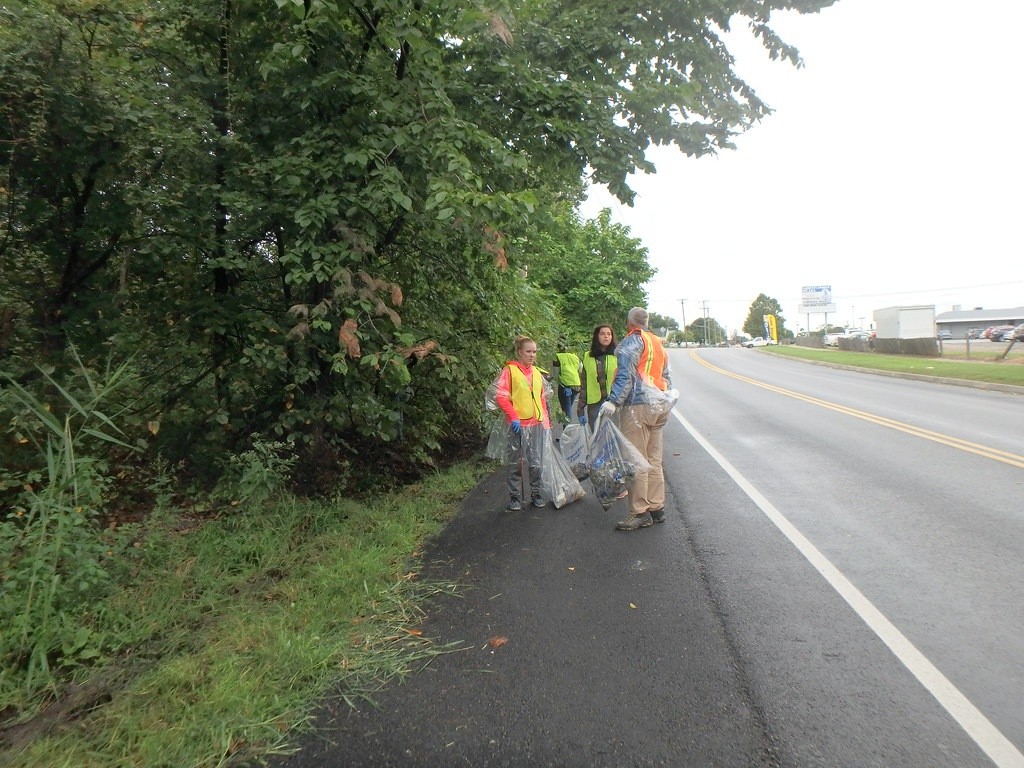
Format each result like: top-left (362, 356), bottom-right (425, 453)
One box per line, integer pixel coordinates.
top-left (967, 322), bottom-right (1024, 342)
top-left (742, 336), bottom-right (771, 348)
top-left (940, 329), bottom-right (953, 340)
top-left (719, 342), bottom-right (730, 348)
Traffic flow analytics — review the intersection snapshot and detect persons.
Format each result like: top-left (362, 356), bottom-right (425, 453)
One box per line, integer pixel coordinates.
top-left (553, 336), bottom-right (583, 442)
top-left (600, 307), bottom-right (672, 530)
top-left (577, 325), bottom-right (617, 432)
top-left (485, 334), bottom-right (551, 510)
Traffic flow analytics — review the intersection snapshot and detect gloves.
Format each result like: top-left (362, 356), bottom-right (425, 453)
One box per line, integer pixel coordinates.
top-left (578, 416), bottom-right (587, 426)
top-left (600, 401), bottom-right (616, 416)
top-left (512, 420), bottom-right (520, 433)
top-left (564, 388), bottom-right (572, 397)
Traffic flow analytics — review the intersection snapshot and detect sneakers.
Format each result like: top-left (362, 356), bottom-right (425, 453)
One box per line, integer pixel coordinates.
top-left (649, 510), bottom-right (666, 523)
top-left (531, 494), bottom-right (545, 507)
top-left (509, 495), bottom-right (521, 510)
top-left (615, 512), bottom-right (653, 531)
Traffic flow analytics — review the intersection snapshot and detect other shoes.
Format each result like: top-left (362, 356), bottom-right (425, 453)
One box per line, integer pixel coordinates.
top-left (556, 438), bottom-right (560, 442)
